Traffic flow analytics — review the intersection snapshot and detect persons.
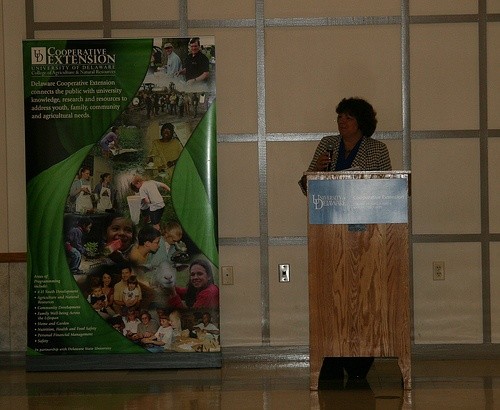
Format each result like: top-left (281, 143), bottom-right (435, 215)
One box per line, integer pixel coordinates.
top-left (175, 38), bottom-right (209, 84)
top-left (200, 45), bottom-right (215, 57)
top-left (138, 92), bottom-right (212, 118)
top-left (130, 183), bottom-right (150, 225)
top-left (149, 123), bottom-right (183, 181)
top-left (101, 126), bottom-right (120, 160)
top-left (87, 260), bottom-right (219, 352)
top-left (132, 176), bottom-right (170, 230)
top-left (70, 168), bottom-right (93, 215)
top-left (103, 214), bottom-right (136, 259)
top-left (145, 222), bottom-right (183, 266)
top-left (163, 43), bottom-right (183, 79)
top-left (93, 173), bottom-right (113, 211)
top-left (298, 98), bottom-right (391, 379)
top-left (128, 227), bottom-right (161, 266)
top-left (65, 218), bottom-right (92, 274)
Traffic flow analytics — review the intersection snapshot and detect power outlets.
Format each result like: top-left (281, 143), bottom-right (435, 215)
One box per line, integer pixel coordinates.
top-left (432, 262), bottom-right (445, 280)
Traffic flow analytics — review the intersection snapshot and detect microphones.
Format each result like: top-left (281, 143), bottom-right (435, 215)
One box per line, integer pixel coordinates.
top-left (326, 143), bottom-right (334, 171)
